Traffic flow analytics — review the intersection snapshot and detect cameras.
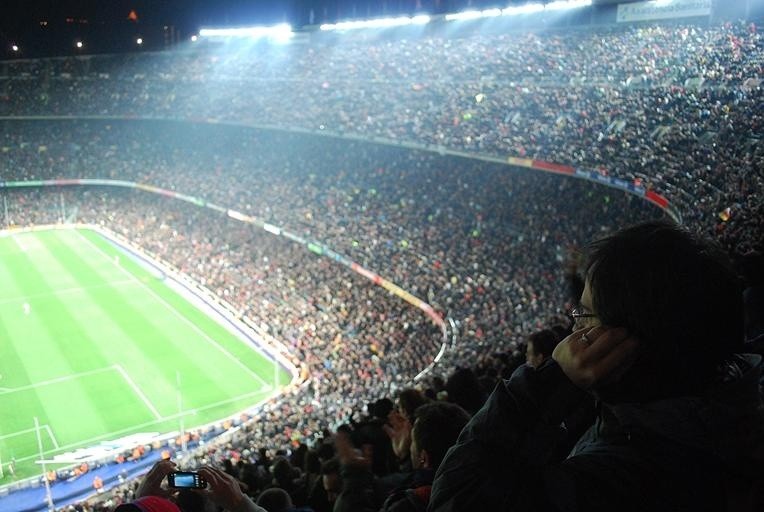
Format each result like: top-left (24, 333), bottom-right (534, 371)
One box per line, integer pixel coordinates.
top-left (167, 470), bottom-right (207, 490)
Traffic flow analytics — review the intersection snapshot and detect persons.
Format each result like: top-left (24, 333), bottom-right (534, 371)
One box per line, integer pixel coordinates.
top-left (290, 17), bottom-right (764, 511)
top-left (110, 15), bottom-right (290, 348)
top-left (43, 394), bottom-right (290, 512)
top-left (2, 16), bottom-right (110, 226)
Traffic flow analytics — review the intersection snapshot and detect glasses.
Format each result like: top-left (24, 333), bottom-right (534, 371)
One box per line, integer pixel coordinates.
top-left (570, 303), bottom-right (597, 330)
